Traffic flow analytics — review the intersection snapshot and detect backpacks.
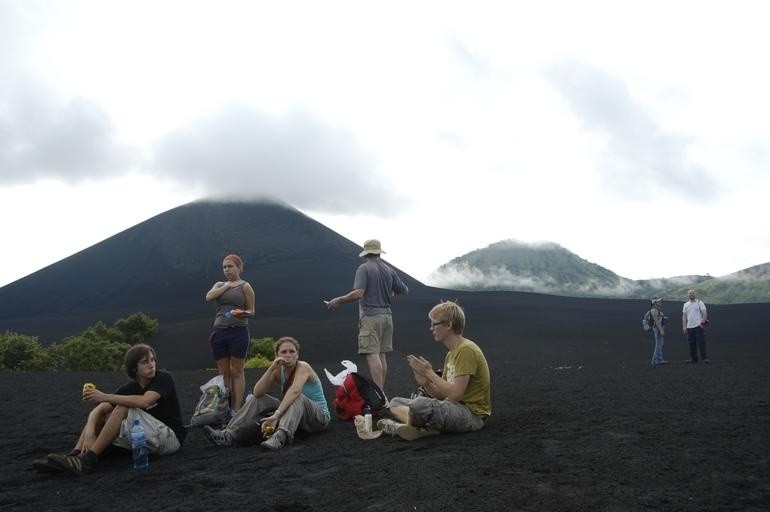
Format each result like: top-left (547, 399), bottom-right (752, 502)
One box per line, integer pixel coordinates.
top-left (186, 386), bottom-right (233, 429)
top-left (642, 307), bottom-right (660, 332)
top-left (410, 368), bottom-right (443, 398)
top-left (334, 371), bottom-right (390, 422)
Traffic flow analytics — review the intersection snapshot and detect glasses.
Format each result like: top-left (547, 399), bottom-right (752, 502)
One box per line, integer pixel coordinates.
top-left (430, 321), bottom-right (447, 327)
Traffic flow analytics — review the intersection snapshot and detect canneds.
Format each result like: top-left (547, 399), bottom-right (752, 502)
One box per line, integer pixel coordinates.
top-left (82, 383), bottom-right (95, 400)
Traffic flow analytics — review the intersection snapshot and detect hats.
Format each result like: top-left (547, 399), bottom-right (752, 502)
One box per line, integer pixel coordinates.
top-left (652, 297), bottom-right (662, 304)
top-left (359, 239), bottom-right (386, 258)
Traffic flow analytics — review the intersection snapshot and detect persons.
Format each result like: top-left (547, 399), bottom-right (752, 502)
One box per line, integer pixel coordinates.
top-left (375, 301), bottom-right (494, 441)
top-left (326, 239), bottom-right (410, 410)
top-left (681, 288), bottom-right (712, 364)
top-left (203, 254), bottom-right (257, 422)
top-left (33, 343), bottom-right (188, 480)
top-left (203, 336), bottom-right (332, 451)
top-left (643, 297), bottom-right (673, 366)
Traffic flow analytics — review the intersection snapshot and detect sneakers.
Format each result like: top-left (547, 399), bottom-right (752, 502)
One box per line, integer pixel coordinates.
top-left (260, 432), bottom-right (287, 450)
top-left (32, 454), bottom-right (75, 477)
top-left (660, 360), bottom-right (669, 364)
top-left (703, 358), bottom-right (709, 362)
top-left (685, 359), bottom-right (696, 364)
top-left (377, 419), bottom-right (407, 437)
top-left (204, 424), bottom-right (235, 448)
top-left (48, 455), bottom-right (89, 478)
top-left (397, 425), bottom-right (440, 441)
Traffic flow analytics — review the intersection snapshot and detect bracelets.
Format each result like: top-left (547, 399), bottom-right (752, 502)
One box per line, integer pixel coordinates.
top-left (275, 410), bottom-right (282, 416)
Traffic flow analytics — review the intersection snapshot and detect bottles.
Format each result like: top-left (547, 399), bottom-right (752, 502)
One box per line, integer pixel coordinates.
top-left (129, 418), bottom-right (151, 471)
top-left (363, 404), bottom-right (373, 433)
